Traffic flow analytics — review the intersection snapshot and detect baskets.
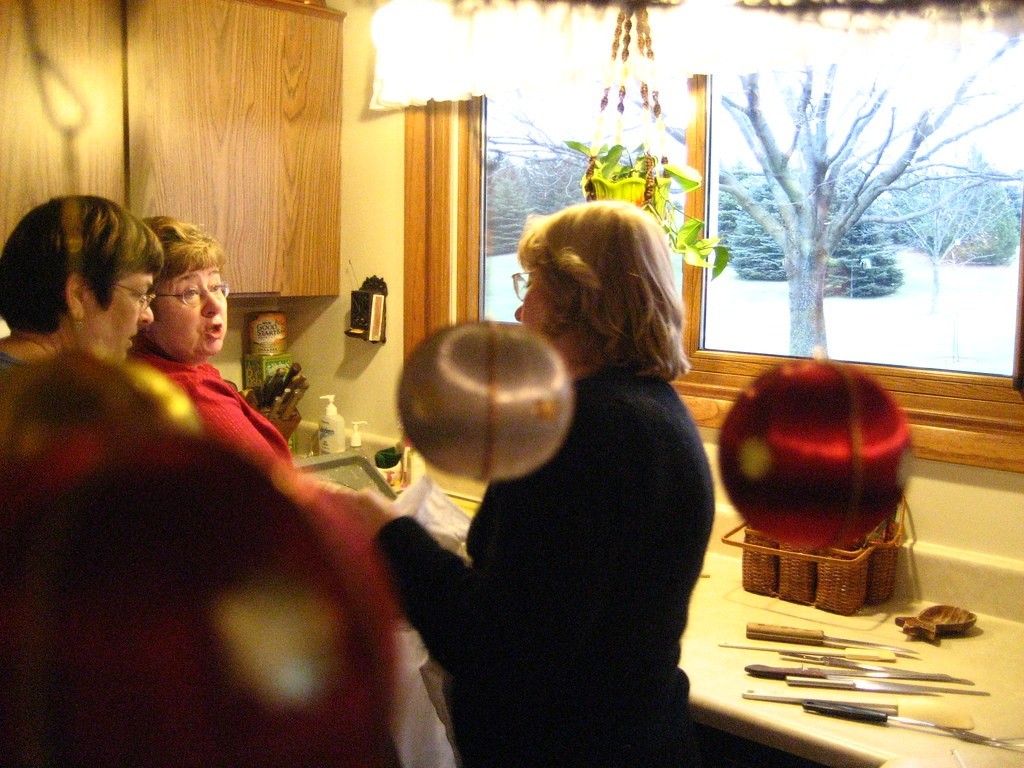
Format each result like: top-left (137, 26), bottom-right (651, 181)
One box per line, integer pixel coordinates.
top-left (721, 360), bottom-right (905, 615)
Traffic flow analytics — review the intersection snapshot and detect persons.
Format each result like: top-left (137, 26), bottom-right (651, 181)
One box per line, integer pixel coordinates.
top-left (0, 193), bottom-right (715, 768)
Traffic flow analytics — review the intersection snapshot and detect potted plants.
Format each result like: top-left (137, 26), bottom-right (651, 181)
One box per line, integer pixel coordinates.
top-left (565, 135), bottom-right (735, 281)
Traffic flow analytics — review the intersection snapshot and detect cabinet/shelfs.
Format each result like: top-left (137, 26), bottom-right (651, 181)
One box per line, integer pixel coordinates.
top-left (1, 1), bottom-right (350, 302)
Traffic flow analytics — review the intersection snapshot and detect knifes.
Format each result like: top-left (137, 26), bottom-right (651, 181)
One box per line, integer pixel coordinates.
top-left (718, 622), bottom-right (1012, 749)
top-left (255, 362), bottom-right (310, 422)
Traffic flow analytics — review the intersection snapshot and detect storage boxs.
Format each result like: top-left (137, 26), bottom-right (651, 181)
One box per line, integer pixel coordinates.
top-left (244, 354), bottom-right (296, 458)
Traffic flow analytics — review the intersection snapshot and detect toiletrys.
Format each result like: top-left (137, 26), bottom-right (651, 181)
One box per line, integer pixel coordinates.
top-left (317, 394), bottom-right (369, 456)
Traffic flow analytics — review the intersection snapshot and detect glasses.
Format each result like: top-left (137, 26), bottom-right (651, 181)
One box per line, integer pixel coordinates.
top-left (111, 283), bottom-right (157, 312)
top-left (513, 270), bottom-right (536, 301)
top-left (154, 283), bottom-right (229, 307)
